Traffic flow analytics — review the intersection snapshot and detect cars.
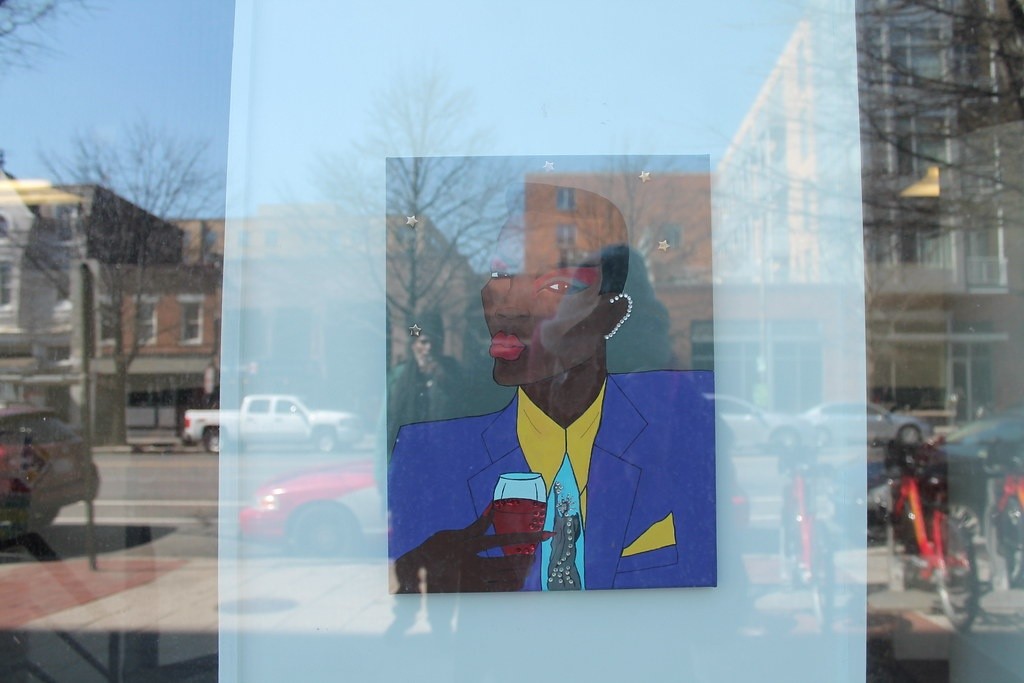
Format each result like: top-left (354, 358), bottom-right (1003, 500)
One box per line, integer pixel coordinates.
top-left (796, 399), bottom-right (935, 448)
top-left (0, 406), bottom-right (100, 530)
top-left (829, 409), bottom-right (1024, 546)
top-left (236, 455), bottom-right (396, 562)
top-left (704, 392), bottom-right (792, 450)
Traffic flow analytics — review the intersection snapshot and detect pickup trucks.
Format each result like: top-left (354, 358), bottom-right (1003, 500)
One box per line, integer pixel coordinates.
top-left (182, 394), bottom-right (362, 454)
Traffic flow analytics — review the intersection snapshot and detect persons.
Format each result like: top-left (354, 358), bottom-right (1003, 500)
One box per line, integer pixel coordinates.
top-left (380, 312), bottom-right (467, 637)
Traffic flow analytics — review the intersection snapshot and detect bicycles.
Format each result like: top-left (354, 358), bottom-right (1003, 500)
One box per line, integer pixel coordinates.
top-left (765, 424), bottom-right (837, 623)
top-left (852, 416), bottom-right (979, 630)
top-left (977, 438), bottom-right (1024, 590)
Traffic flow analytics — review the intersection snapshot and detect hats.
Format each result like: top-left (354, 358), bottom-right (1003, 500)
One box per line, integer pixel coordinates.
top-left (408, 311), bottom-right (444, 343)
